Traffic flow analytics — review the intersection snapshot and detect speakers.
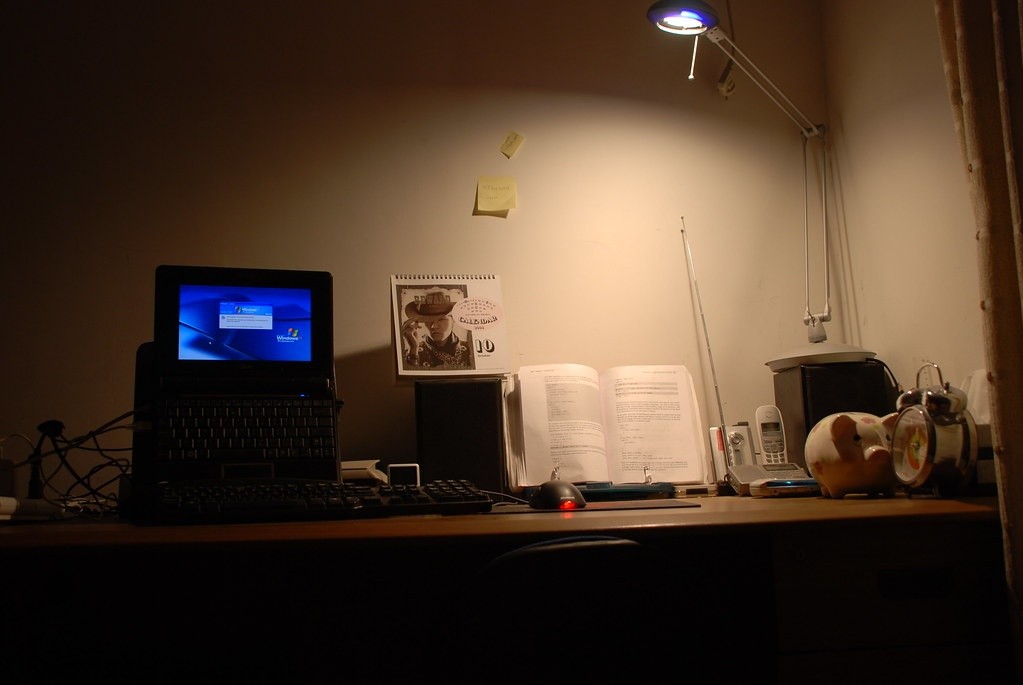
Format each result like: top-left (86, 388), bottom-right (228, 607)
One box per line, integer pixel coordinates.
top-left (771, 361), bottom-right (888, 480)
top-left (413, 379), bottom-right (504, 504)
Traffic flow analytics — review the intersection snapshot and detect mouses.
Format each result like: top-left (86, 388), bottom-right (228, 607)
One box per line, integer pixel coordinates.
top-left (528, 480), bottom-right (588, 510)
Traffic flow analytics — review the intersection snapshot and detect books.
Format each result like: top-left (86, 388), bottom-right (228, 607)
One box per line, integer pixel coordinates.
top-left (502, 366), bottom-right (707, 493)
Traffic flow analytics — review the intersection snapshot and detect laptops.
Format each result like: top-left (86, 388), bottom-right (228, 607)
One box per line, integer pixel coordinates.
top-left (151, 261), bottom-right (339, 480)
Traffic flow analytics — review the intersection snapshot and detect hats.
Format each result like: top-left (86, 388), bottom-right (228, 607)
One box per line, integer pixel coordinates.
top-left (405, 287), bottom-right (457, 323)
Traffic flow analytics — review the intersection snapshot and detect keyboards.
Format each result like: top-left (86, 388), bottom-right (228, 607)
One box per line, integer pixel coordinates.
top-left (152, 475), bottom-right (494, 522)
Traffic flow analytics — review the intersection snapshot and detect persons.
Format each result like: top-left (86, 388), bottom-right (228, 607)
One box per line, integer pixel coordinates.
top-left (402, 292), bottom-right (470, 367)
